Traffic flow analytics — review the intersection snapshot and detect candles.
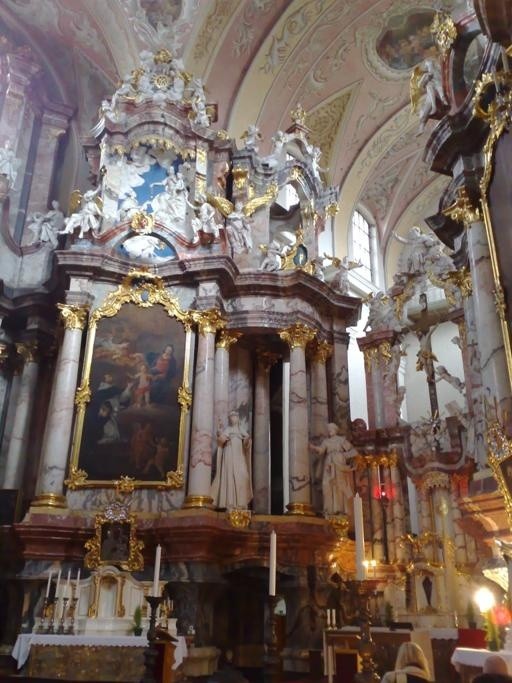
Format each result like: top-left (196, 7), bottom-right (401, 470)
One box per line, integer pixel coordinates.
top-left (352, 491), bottom-right (369, 579)
top-left (324, 607), bottom-right (338, 627)
top-left (269, 525), bottom-right (278, 597)
top-left (404, 473), bottom-right (423, 538)
top-left (150, 541), bottom-right (163, 598)
top-left (41, 565), bottom-right (84, 600)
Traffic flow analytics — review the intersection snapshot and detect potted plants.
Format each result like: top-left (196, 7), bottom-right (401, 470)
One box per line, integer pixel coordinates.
top-left (465, 596), bottom-right (500, 652)
top-left (383, 602), bottom-right (396, 632)
top-left (131, 605), bottom-right (144, 636)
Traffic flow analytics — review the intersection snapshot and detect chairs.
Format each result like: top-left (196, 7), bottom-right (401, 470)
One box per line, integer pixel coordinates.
top-left (281, 624), bottom-right (512, 682)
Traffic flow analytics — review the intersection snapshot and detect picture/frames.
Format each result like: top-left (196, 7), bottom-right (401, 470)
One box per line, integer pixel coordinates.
top-left (68, 264), bottom-right (194, 489)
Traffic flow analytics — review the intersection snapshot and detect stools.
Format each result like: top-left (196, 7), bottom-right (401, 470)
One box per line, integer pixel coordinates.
top-left (186, 644), bottom-right (223, 676)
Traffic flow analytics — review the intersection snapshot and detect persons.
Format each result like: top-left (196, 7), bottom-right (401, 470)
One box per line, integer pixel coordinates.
top-left (25, 45), bottom-right (354, 297)
top-left (0, 139), bottom-right (19, 192)
top-left (96, 330), bottom-right (179, 476)
top-left (209, 410), bottom-right (254, 507)
top-left (416, 57), bottom-right (448, 137)
top-left (362, 225), bottom-right (482, 402)
top-left (308, 418), bottom-right (361, 516)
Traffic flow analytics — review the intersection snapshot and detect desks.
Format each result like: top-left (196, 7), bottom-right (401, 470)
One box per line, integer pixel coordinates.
top-left (16, 632), bottom-right (184, 683)
